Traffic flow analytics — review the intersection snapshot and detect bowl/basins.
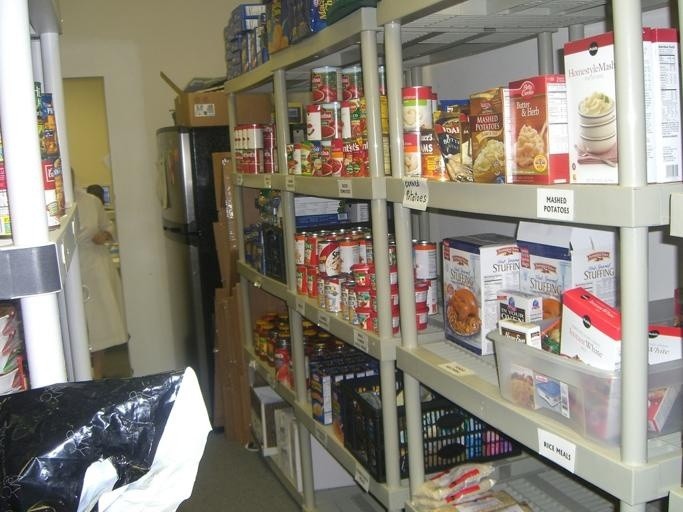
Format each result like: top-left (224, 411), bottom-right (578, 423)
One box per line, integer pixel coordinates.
top-left (578, 98), bottom-right (616, 155)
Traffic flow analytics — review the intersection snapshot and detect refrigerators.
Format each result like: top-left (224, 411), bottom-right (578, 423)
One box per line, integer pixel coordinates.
top-left (151, 126), bottom-right (230, 433)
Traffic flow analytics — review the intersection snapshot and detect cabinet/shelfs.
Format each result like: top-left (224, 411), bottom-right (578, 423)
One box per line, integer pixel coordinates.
top-left (222, 0), bottom-right (559, 511)
top-left (1, 1), bottom-right (98, 398)
top-left (377, 0), bottom-right (681, 512)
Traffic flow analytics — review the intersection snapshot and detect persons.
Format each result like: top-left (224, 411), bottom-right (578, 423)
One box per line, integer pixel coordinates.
top-left (69, 166), bottom-right (134, 381)
top-left (85, 184), bottom-right (105, 207)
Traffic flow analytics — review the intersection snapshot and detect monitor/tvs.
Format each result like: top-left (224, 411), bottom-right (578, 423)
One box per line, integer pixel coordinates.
top-left (80, 186), bottom-right (110, 211)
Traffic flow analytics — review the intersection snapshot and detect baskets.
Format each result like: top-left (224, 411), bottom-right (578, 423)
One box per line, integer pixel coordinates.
top-left (337, 371), bottom-right (523, 485)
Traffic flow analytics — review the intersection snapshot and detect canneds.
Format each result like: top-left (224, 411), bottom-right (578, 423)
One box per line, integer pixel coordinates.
top-left (0, 80), bottom-right (66, 398)
top-left (234, 63), bottom-right (438, 392)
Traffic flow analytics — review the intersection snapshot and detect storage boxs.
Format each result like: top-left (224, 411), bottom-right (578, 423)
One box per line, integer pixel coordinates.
top-left (159, 71), bottom-right (272, 125)
